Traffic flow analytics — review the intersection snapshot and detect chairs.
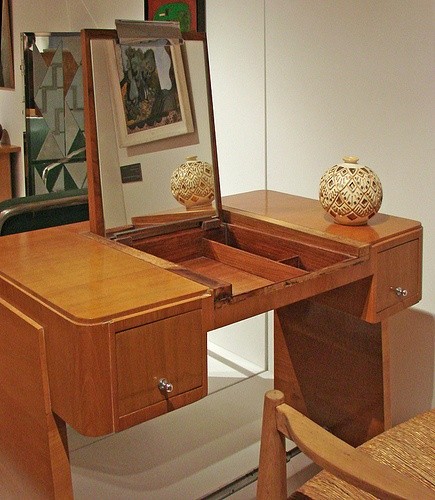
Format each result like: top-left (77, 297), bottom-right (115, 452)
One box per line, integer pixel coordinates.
top-left (256, 390), bottom-right (435, 500)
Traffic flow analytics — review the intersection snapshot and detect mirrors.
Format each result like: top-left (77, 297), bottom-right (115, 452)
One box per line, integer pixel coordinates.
top-left (80, 27), bottom-right (224, 236)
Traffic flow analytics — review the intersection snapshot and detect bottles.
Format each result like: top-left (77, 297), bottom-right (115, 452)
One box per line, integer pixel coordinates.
top-left (318, 156), bottom-right (383, 225)
top-left (170, 155), bottom-right (215, 211)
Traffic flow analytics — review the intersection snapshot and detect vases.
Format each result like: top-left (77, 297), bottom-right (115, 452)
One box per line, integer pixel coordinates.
top-left (319, 155), bottom-right (383, 226)
top-left (171, 155), bottom-right (216, 211)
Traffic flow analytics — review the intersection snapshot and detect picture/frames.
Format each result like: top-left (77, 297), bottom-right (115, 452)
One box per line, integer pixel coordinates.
top-left (143, 0), bottom-right (205, 33)
top-left (101, 39), bottom-right (194, 148)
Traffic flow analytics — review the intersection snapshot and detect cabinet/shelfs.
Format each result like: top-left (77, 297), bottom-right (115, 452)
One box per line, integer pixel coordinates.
top-left (0, 187), bottom-right (424, 500)
